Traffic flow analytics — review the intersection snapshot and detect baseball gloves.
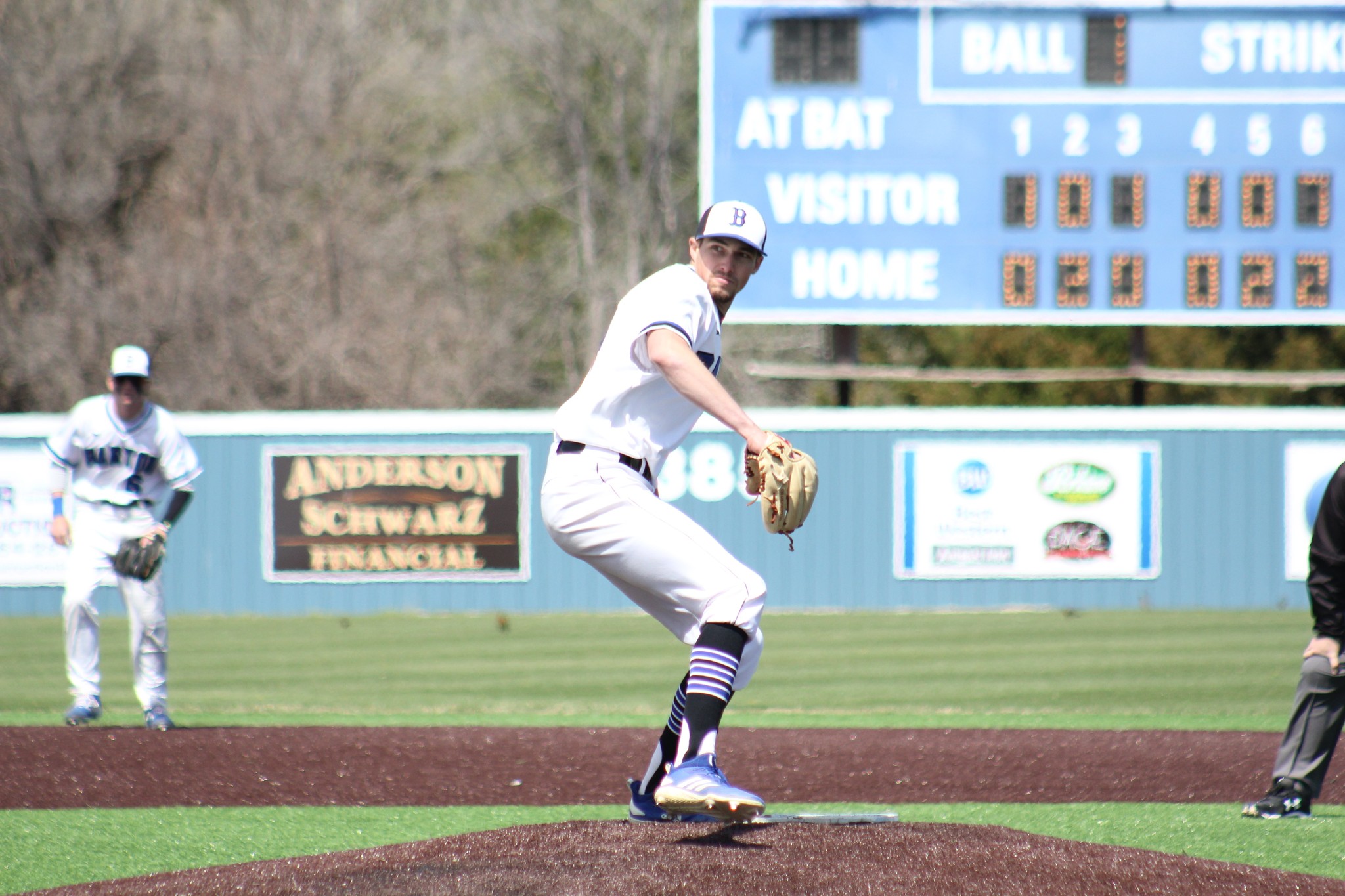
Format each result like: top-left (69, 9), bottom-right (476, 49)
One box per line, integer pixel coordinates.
top-left (743, 429), bottom-right (819, 554)
top-left (102, 534), bottom-right (167, 584)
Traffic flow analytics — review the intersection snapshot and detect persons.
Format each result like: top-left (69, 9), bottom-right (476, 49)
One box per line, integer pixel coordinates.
top-left (1243, 461), bottom-right (1345, 818)
top-left (45, 346), bottom-right (202, 728)
top-left (541, 198), bottom-right (818, 823)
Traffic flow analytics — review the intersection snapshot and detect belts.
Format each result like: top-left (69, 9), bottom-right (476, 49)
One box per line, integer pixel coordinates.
top-left (556, 441), bottom-right (652, 480)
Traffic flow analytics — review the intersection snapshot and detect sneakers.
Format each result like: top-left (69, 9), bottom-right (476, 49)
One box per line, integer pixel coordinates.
top-left (1242, 777), bottom-right (1312, 819)
top-left (65, 695), bottom-right (101, 725)
top-left (627, 779), bottom-right (730, 823)
top-left (145, 708), bottom-right (175, 730)
top-left (655, 753), bottom-right (765, 824)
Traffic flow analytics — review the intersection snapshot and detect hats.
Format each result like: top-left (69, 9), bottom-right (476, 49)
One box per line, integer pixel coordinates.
top-left (696, 201), bottom-right (768, 257)
top-left (111, 345), bottom-right (150, 377)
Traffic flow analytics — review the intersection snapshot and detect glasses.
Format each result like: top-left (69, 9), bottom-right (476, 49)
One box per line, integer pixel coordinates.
top-left (116, 376), bottom-right (141, 386)
